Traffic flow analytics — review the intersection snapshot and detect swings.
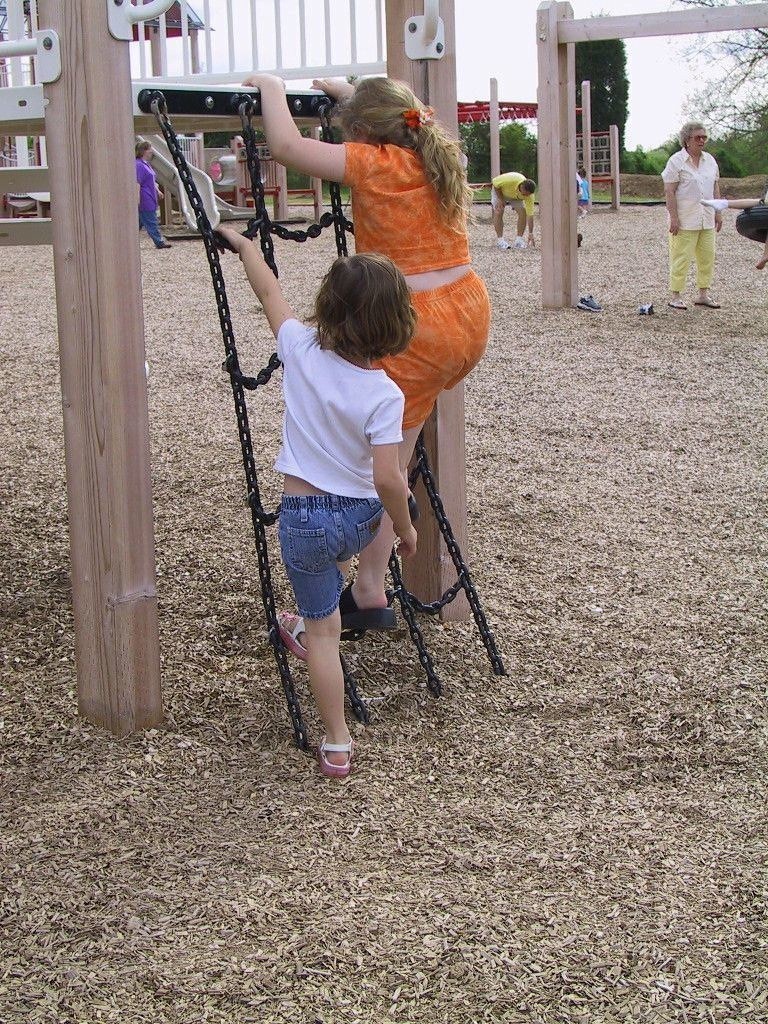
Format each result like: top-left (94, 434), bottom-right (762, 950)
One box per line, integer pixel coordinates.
top-left (736, 193), bottom-right (768, 242)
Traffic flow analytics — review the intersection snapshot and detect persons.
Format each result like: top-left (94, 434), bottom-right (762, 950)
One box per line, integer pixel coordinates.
top-left (458, 149), bottom-right (592, 250)
top-left (211, 227), bottom-right (417, 778)
top-left (663, 121), bottom-right (768, 310)
top-left (243, 73), bottom-right (488, 633)
top-left (136, 141), bottom-right (174, 249)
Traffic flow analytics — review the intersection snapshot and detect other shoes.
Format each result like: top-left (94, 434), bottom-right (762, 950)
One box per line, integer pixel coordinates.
top-left (514, 240), bottom-right (527, 249)
top-left (156, 244), bottom-right (172, 249)
top-left (577, 234), bottom-right (583, 248)
top-left (496, 240), bottom-right (511, 249)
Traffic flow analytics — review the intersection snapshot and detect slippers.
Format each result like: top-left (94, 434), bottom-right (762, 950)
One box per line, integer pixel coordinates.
top-left (695, 297), bottom-right (721, 309)
top-left (668, 300), bottom-right (687, 311)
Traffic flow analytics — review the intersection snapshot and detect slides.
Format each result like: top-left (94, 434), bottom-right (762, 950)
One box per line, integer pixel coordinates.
top-left (136, 135), bottom-right (221, 232)
top-left (210, 189), bottom-right (258, 219)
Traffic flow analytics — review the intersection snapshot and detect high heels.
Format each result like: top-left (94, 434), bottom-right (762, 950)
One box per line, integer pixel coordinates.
top-left (339, 579), bottom-right (393, 629)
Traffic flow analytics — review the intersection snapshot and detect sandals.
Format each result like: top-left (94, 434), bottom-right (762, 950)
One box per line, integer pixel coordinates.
top-left (276, 612), bottom-right (308, 663)
top-left (318, 735), bottom-right (355, 778)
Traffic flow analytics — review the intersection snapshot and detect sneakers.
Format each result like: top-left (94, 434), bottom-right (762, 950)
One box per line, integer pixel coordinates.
top-left (640, 303), bottom-right (654, 315)
top-left (577, 295), bottom-right (604, 312)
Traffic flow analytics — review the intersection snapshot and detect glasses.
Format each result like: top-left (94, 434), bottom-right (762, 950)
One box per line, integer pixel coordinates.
top-left (689, 135), bottom-right (708, 143)
